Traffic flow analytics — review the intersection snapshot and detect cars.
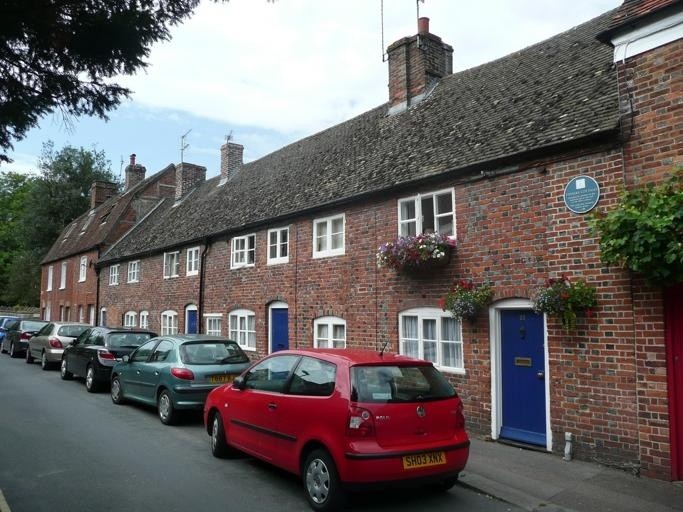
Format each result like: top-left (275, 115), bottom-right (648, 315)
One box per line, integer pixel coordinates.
top-left (1, 314), bottom-right (47, 360)
top-left (0, 316), bottom-right (19, 343)
top-left (201, 347), bottom-right (473, 512)
top-left (104, 329), bottom-right (253, 426)
top-left (57, 324), bottom-right (158, 392)
top-left (21, 319), bottom-right (99, 374)
